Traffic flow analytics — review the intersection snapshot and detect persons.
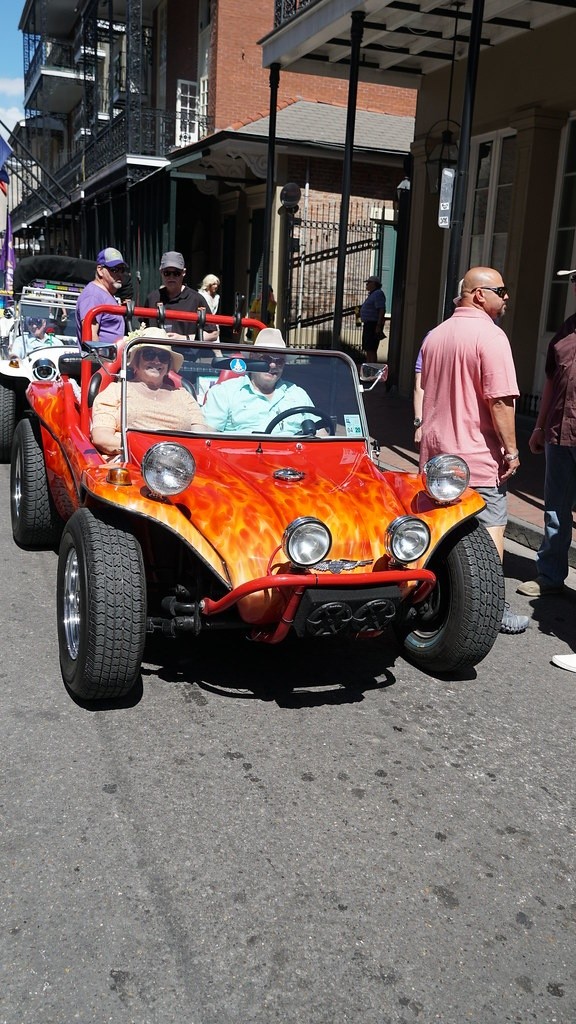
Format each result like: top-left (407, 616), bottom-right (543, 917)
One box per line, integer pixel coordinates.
top-left (92, 326), bottom-right (206, 464)
top-left (360, 276), bottom-right (386, 363)
top-left (75, 248), bottom-right (129, 354)
top-left (138, 252), bottom-right (219, 358)
top-left (250, 286), bottom-right (276, 343)
top-left (517, 269), bottom-right (576, 673)
top-left (40, 294), bottom-right (68, 335)
top-left (414, 280), bottom-right (464, 452)
top-left (11, 316), bottom-right (64, 359)
top-left (204, 328), bottom-right (327, 436)
top-left (418, 267), bottom-right (519, 564)
top-left (198, 274), bottom-right (220, 314)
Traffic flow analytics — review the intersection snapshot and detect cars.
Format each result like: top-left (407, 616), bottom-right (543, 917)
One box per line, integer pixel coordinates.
top-left (10, 298), bottom-right (506, 707)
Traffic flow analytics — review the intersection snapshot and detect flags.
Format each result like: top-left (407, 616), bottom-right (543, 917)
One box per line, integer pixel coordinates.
top-left (0, 216), bottom-right (16, 303)
top-left (0, 134), bottom-right (14, 196)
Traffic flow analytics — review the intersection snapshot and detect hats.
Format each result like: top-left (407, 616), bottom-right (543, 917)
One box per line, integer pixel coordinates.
top-left (363, 276), bottom-right (381, 284)
top-left (453, 279), bottom-right (464, 305)
top-left (557, 269), bottom-right (576, 276)
top-left (126, 322), bottom-right (184, 373)
top-left (97, 247), bottom-right (129, 268)
top-left (241, 327), bottom-right (300, 362)
top-left (159, 251), bottom-right (185, 270)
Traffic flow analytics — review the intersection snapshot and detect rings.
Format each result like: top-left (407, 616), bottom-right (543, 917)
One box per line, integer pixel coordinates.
top-left (512, 470), bottom-right (516, 475)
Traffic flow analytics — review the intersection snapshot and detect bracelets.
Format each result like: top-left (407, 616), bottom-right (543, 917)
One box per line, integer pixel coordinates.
top-left (503, 449), bottom-right (519, 461)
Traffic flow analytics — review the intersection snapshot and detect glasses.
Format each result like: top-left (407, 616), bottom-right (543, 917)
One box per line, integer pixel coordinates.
top-left (27, 315), bottom-right (43, 329)
top-left (471, 286), bottom-right (509, 298)
top-left (260, 355), bottom-right (286, 366)
top-left (143, 349), bottom-right (172, 364)
top-left (109, 266), bottom-right (126, 273)
top-left (571, 274), bottom-right (576, 284)
top-left (164, 270), bottom-right (181, 277)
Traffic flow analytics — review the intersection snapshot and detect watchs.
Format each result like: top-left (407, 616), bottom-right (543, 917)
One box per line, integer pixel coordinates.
top-left (414, 418), bottom-right (422, 427)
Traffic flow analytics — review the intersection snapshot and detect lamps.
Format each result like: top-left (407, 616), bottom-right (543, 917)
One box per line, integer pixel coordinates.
top-left (426, 0), bottom-right (467, 193)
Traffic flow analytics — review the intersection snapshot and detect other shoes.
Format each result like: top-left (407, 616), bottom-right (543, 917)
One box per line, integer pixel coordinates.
top-left (517, 577), bottom-right (564, 595)
top-left (552, 653), bottom-right (576, 672)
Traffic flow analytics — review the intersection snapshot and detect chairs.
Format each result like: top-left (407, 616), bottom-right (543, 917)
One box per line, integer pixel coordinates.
top-left (87, 337), bottom-right (198, 414)
top-left (57, 352), bottom-right (221, 386)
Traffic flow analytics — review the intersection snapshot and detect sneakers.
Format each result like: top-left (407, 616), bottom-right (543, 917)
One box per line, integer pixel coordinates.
top-left (500, 602), bottom-right (530, 633)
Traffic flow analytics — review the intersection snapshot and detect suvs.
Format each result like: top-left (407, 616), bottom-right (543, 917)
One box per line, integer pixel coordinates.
top-left (0, 254), bottom-right (133, 465)
